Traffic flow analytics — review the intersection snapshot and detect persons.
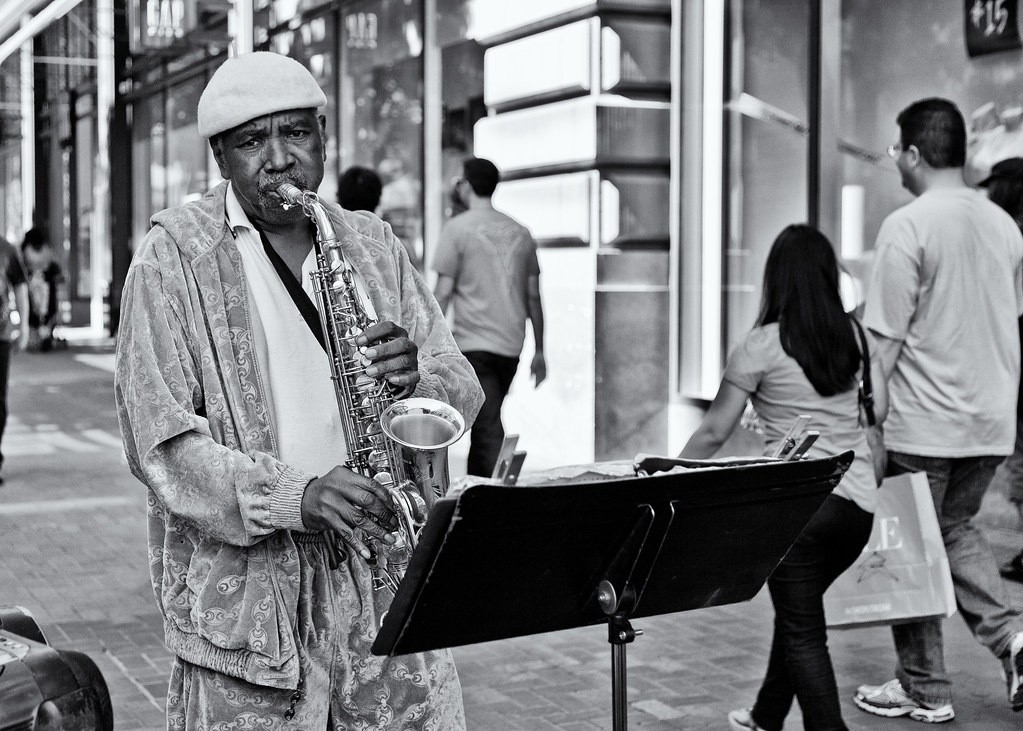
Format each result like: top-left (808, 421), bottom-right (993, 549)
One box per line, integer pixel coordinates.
top-left (0, 236), bottom-right (29, 484)
top-left (853, 98), bottom-right (1023, 723)
top-left (968, 157), bottom-right (1023, 584)
top-left (338, 164), bottom-right (385, 211)
top-left (21, 208), bottom-right (71, 353)
top-left (113, 49), bottom-right (484, 731)
top-left (430, 158), bottom-right (547, 480)
top-left (677, 224), bottom-right (889, 731)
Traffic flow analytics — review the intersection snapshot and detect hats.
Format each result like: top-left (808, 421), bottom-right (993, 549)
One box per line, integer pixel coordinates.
top-left (197, 52), bottom-right (328, 138)
top-left (975, 156), bottom-right (1023, 187)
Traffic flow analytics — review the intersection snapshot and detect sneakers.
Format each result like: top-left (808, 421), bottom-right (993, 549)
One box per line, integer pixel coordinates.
top-left (729, 707), bottom-right (771, 731)
top-left (1000, 632), bottom-right (1022, 712)
top-left (853, 677), bottom-right (956, 723)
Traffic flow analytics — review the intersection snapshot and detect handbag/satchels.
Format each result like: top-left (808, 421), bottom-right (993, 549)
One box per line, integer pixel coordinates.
top-left (823, 474), bottom-right (955, 628)
top-left (848, 311), bottom-right (888, 487)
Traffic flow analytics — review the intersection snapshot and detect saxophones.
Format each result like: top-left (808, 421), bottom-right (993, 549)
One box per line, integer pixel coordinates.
top-left (276, 181), bottom-right (466, 597)
